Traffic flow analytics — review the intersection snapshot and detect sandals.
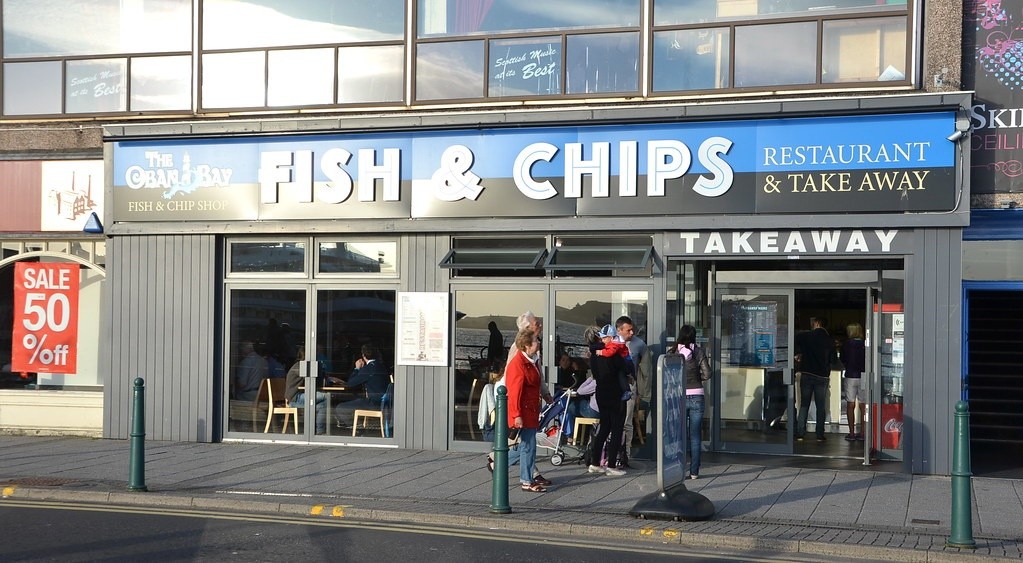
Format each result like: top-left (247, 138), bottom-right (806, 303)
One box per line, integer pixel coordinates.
top-left (486, 463), bottom-right (494, 473)
top-left (522, 482), bottom-right (547, 493)
top-left (487, 455), bottom-right (494, 462)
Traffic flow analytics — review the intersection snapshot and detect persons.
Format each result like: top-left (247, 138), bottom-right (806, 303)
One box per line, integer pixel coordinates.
top-left (559, 355), bottom-right (591, 391)
top-left (268, 318), bottom-right (297, 365)
top-left (236, 340), bottom-right (269, 390)
top-left (487, 315), bottom-right (555, 484)
top-left (487, 320), bottom-right (504, 373)
top-left (668, 325), bottom-right (712, 479)
top-left (477, 366), bottom-right (507, 442)
top-left (559, 373), bottom-right (600, 446)
top-left (794, 315), bottom-right (833, 441)
top-left (284, 347), bottom-right (335, 435)
top-left (584, 316), bottom-right (651, 476)
top-left (487, 329), bottom-right (547, 492)
top-left (838, 323), bottom-right (865, 441)
top-left (335, 344), bottom-right (387, 437)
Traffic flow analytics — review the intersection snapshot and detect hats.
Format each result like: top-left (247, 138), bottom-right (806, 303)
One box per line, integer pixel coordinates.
top-left (598, 324), bottom-right (617, 338)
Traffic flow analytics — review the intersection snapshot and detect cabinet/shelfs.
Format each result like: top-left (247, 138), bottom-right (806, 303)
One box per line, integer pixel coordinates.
top-left (872, 304), bottom-right (904, 451)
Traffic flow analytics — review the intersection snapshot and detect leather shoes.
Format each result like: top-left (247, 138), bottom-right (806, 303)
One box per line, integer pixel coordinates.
top-left (533, 474), bottom-right (551, 485)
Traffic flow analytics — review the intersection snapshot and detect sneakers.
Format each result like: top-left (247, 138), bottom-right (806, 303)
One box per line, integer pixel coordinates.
top-left (844, 434), bottom-right (854, 440)
top-left (621, 391), bottom-right (633, 401)
top-left (588, 464), bottom-right (605, 473)
top-left (856, 435), bottom-right (864, 441)
top-left (605, 466), bottom-right (627, 476)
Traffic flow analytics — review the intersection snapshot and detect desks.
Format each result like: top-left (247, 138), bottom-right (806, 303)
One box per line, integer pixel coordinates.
top-left (298, 386), bottom-right (352, 436)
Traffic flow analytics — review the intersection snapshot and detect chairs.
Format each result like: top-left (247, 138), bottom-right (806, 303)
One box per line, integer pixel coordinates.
top-left (455, 379), bottom-right (486, 439)
top-left (573, 396), bottom-right (645, 445)
top-left (230, 378), bottom-right (304, 435)
top-left (352, 383), bottom-right (394, 438)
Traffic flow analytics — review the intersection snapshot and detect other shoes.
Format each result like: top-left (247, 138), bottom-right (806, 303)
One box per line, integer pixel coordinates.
top-left (816, 437), bottom-right (826, 441)
top-left (794, 435), bottom-right (803, 441)
